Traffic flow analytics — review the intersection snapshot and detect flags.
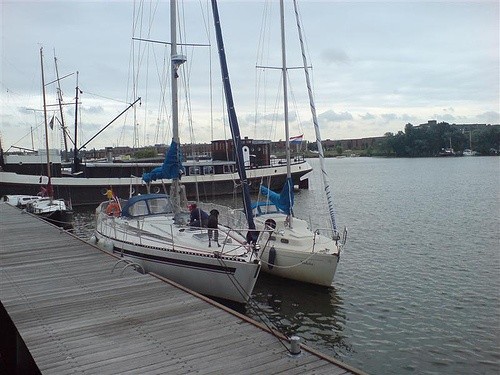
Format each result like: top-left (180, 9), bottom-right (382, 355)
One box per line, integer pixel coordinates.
top-left (288, 134), bottom-right (304, 145)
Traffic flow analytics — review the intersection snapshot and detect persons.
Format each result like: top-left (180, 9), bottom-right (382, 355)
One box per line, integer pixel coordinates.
top-left (103, 187), bottom-right (113, 201)
top-left (188, 204), bottom-right (204, 227)
top-left (207, 209), bottom-right (222, 248)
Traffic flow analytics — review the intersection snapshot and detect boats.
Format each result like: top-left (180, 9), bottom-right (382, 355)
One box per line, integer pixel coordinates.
top-left (0, 136), bottom-right (315, 207)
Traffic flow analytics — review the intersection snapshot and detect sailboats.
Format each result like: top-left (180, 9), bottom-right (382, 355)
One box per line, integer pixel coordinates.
top-left (90, 0), bottom-right (275, 304)
top-left (234, 0), bottom-right (348, 288)
top-left (4, 47), bottom-right (77, 235)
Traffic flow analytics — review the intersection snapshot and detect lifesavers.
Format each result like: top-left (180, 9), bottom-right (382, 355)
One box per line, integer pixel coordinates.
top-left (105, 202), bottom-right (121, 218)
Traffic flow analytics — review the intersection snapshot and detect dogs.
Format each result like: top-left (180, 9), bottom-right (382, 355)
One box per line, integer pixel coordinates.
top-left (207, 209), bottom-right (221, 247)
